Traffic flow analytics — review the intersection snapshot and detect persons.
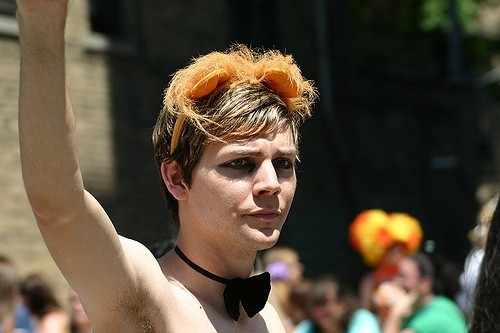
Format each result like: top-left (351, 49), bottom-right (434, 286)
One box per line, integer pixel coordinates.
top-left (15, 0), bottom-right (319, 333)
top-left (0, 196), bottom-right (500, 333)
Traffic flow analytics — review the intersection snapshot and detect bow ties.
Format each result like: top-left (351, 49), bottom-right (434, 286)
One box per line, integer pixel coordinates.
top-left (175, 245), bottom-right (272, 322)
